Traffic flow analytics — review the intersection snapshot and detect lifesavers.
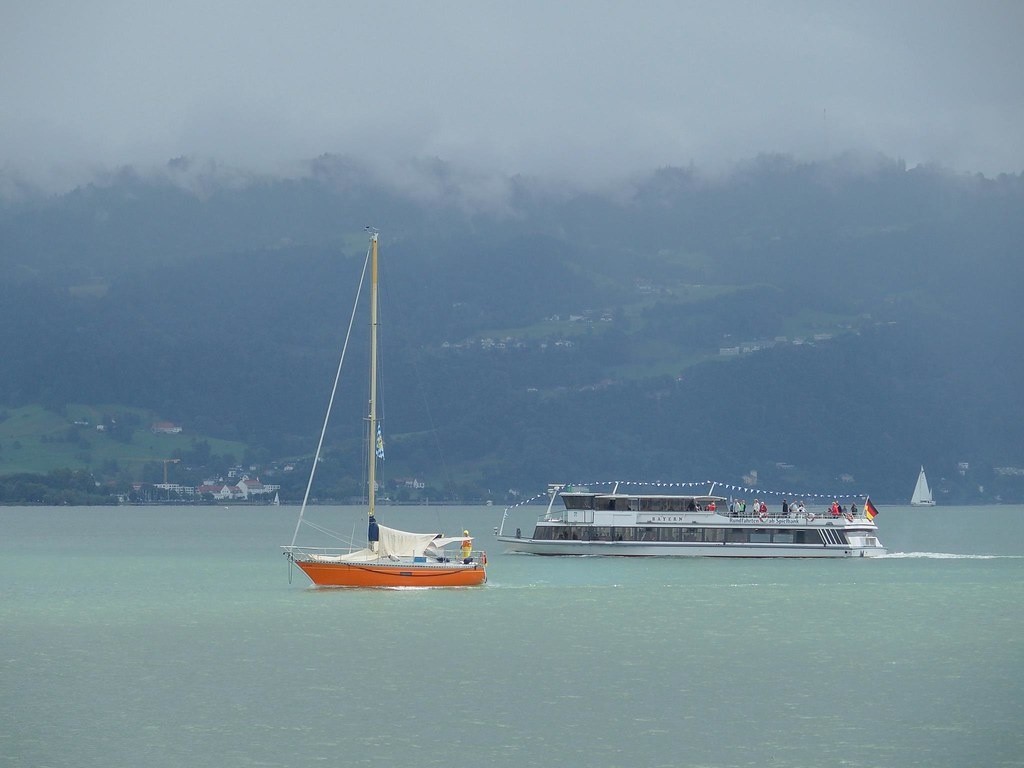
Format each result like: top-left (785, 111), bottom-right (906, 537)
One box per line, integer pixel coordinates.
top-left (759, 512), bottom-right (768, 523)
top-left (650, 516), bottom-right (684, 523)
top-left (806, 513), bottom-right (814, 521)
top-left (847, 513), bottom-right (854, 522)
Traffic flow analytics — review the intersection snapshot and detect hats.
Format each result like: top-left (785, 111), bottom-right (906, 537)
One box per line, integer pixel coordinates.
top-left (464, 530), bottom-right (468, 534)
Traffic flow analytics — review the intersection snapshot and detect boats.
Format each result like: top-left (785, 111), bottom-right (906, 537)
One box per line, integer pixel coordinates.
top-left (494, 481), bottom-right (889, 559)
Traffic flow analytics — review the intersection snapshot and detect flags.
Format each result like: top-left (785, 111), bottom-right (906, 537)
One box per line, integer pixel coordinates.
top-left (864, 498), bottom-right (879, 522)
top-left (375, 423), bottom-right (385, 461)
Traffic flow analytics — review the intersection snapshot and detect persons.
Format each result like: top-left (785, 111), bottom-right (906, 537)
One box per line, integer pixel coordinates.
top-left (596, 532), bottom-right (622, 541)
top-left (687, 501), bottom-right (702, 511)
top-left (843, 505), bottom-right (847, 516)
top-left (460, 530), bottom-right (472, 560)
top-left (783, 499), bottom-right (788, 518)
top-left (850, 503), bottom-right (857, 517)
top-left (789, 500), bottom-right (804, 519)
top-left (559, 531), bottom-right (577, 540)
top-left (704, 502), bottom-right (718, 512)
top-left (516, 528), bottom-right (521, 538)
top-left (760, 502), bottom-right (767, 517)
top-left (730, 499), bottom-right (746, 517)
top-left (753, 499), bottom-right (759, 517)
top-left (828, 501), bottom-right (841, 518)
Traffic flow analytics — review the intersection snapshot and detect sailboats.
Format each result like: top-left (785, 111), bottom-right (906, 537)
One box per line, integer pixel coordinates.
top-left (911, 465), bottom-right (936, 506)
top-left (280, 226), bottom-right (488, 589)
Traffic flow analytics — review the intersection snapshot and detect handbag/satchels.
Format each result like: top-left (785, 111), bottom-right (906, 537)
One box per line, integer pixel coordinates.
top-left (464, 556), bottom-right (473, 564)
top-left (437, 557), bottom-right (450, 562)
